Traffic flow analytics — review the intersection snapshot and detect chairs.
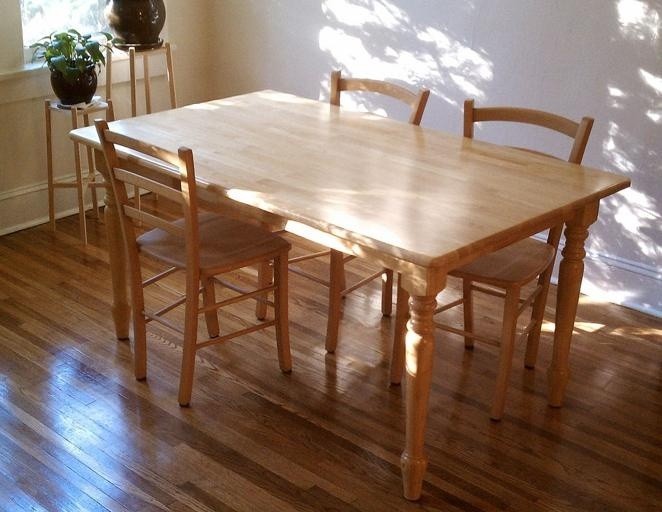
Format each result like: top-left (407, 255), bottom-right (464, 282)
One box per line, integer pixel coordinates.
top-left (390, 98), bottom-right (596, 420)
top-left (95, 119), bottom-right (294, 401)
top-left (254, 71), bottom-right (432, 352)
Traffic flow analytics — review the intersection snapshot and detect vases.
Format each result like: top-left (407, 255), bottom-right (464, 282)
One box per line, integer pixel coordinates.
top-left (104, 0), bottom-right (167, 52)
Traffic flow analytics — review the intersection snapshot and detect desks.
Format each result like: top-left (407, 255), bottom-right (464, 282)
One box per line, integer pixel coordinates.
top-left (43, 43), bottom-right (178, 244)
top-left (67, 89), bottom-right (633, 501)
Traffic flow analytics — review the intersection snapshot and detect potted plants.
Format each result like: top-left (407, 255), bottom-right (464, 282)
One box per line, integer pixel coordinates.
top-left (27, 29), bottom-right (126, 105)
